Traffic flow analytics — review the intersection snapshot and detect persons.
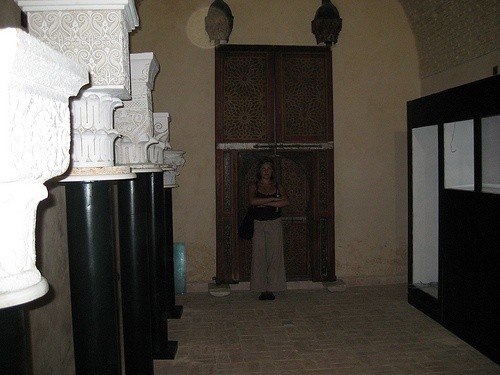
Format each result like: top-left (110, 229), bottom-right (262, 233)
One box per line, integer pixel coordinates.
top-left (248, 158), bottom-right (290, 300)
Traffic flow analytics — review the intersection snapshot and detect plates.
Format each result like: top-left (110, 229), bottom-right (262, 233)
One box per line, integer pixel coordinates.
top-left (210, 291), bottom-right (231, 296)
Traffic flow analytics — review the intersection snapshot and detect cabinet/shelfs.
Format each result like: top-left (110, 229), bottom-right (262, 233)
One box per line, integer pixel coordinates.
top-left (408, 74), bottom-right (499, 368)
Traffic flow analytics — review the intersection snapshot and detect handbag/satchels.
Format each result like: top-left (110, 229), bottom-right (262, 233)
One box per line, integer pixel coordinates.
top-left (236, 182), bottom-right (259, 240)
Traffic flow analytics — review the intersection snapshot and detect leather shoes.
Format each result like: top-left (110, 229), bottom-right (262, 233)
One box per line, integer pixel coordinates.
top-left (259, 292), bottom-right (276, 301)
